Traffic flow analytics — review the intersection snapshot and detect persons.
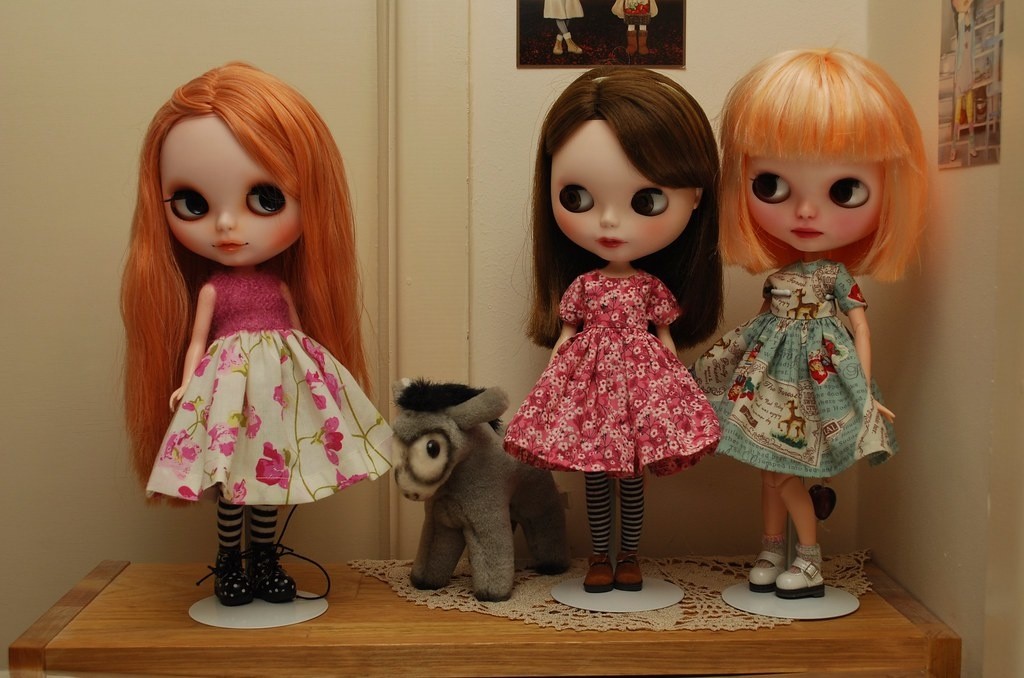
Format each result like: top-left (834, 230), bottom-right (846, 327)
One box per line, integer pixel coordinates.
top-left (502, 64), bottom-right (723, 593)
top-left (118, 63), bottom-right (396, 611)
top-left (686, 46), bottom-right (924, 600)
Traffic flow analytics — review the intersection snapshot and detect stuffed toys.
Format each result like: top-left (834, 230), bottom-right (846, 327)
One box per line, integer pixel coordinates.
top-left (389, 375), bottom-right (568, 603)
